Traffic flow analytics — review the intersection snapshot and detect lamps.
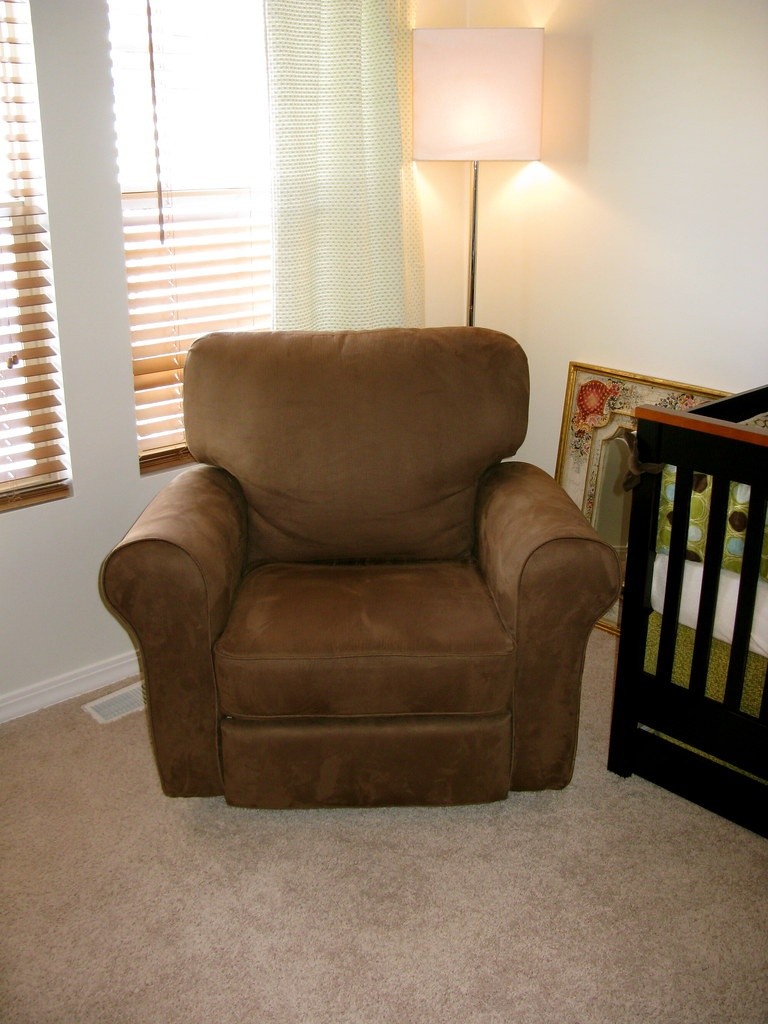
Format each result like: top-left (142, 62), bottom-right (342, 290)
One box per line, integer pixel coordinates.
top-left (412, 28), bottom-right (544, 326)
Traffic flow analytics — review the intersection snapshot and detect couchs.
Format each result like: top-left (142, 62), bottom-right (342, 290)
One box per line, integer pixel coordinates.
top-left (96, 327), bottom-right (631, 809)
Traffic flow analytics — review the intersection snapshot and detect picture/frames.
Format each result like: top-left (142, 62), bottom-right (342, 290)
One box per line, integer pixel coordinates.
top-left (553, 362), bottom-right (736, 637)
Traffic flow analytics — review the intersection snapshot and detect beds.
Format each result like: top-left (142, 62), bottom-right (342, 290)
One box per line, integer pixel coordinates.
top-left (608, 384), bottom-right (768, 841)
